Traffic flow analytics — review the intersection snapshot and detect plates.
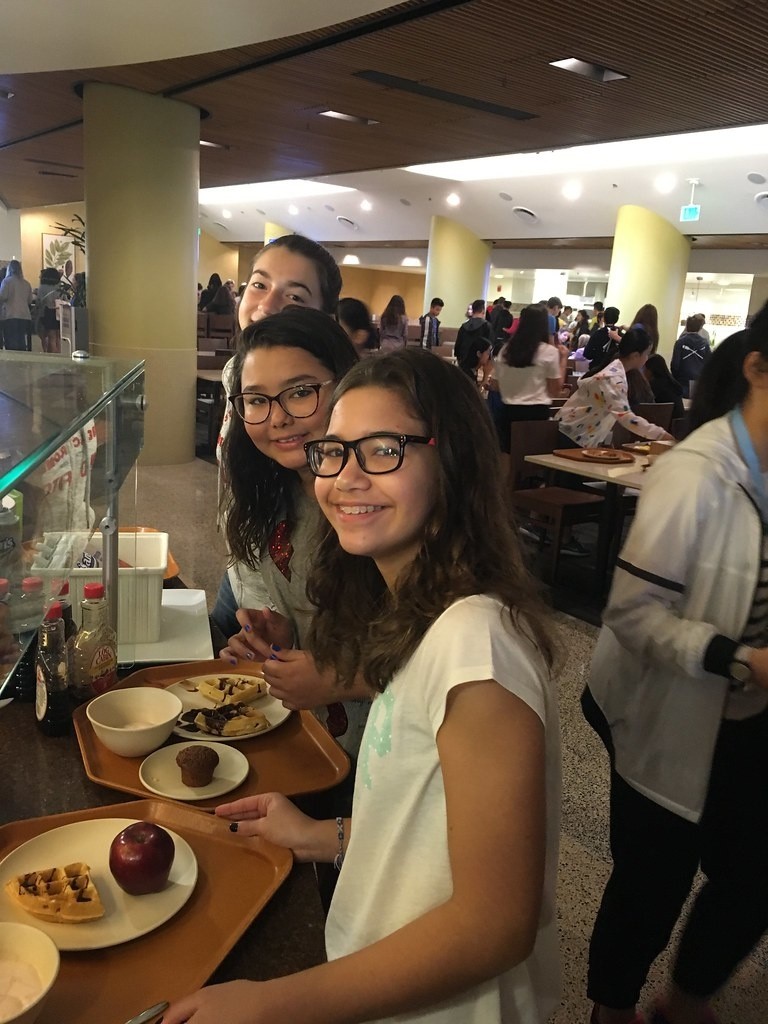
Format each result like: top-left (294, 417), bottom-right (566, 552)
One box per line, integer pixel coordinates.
top-left (0, 818), bottom-right (198, 950)
top-left (582, 450), bottom-right (620, 459)
top-left (138, 741), bottom-right (249, 799)
top-left (164, 674), bottom-right (291, 741)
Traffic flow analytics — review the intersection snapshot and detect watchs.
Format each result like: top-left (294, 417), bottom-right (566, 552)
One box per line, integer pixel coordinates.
top-left (729, 645), bottom-right (752, 689)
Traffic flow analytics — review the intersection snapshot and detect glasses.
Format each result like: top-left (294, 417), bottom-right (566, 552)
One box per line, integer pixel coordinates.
top-left (304, 432), bottom-right (438, 478)
top-left (228, 378), bottom-right (338, 425)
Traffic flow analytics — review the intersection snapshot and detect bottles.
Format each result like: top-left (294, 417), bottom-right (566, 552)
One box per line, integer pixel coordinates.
top-left (0, 502), bottom-right (117, 737)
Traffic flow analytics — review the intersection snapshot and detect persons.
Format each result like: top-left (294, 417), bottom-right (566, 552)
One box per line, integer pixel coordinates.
top-left (219, 307), bottom-right (396, 923)
top-left (334, 296), bottom-right (716, 557)
top-left (0, 260), bottom-right (86, 352)
top-left (163, 347), bottom-right (564, 1024)
top-left (581, 300), bottom-right (768, 1024)
top-left (208, 235), bottom-right (349, 641)
top-left (198, 273), bottom-right (248, 314)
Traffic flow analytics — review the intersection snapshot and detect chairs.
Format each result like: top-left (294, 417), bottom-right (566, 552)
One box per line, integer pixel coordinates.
top-left (196, 295), bottom-right (675, 571)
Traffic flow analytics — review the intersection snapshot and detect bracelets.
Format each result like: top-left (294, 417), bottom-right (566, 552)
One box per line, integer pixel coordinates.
top-left (333, 816), bottom-right (346, 871)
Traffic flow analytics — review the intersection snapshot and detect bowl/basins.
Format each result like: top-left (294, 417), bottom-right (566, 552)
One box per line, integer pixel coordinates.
top-left (0, 922), bottom-right (60, 1024)
top-left (86, 687), bottom-right (182, 757)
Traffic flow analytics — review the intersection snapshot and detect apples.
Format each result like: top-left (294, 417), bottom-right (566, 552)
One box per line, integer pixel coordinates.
top-left (108, 822), bottom-right (174, 896)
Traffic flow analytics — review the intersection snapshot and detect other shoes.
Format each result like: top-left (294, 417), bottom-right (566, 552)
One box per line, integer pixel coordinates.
top-left (655, 1000), bottom-right (722, 1024)
top-left (559, 536), bottom-right (592, 555)
top-left (591, 1004), bottom-right (648, 1024)
top-left (518, 522), bottom-right (553, 545)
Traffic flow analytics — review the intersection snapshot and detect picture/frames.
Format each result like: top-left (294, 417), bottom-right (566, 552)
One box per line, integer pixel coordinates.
top-left (42, 234), bottom-right (76, 287)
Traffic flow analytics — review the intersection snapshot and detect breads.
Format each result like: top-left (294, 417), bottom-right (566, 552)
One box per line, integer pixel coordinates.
top-left (4, 863), bottom-right (105, 924)
top-left (199, 677), bottom-right (268, 706)
top-left (194, 701), bottom-right (271, 736)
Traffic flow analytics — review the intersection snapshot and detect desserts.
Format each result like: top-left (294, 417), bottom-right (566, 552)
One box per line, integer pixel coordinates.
top-left (176, 745), bottom-right (219, 787)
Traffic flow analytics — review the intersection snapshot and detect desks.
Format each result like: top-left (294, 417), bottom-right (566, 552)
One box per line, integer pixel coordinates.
top-left (523, 448), bottom-right (655, 629)
top-left (196, 367), bottom-right (222, 447)
top-left (0, 476), bottom-right (357, 1024)
